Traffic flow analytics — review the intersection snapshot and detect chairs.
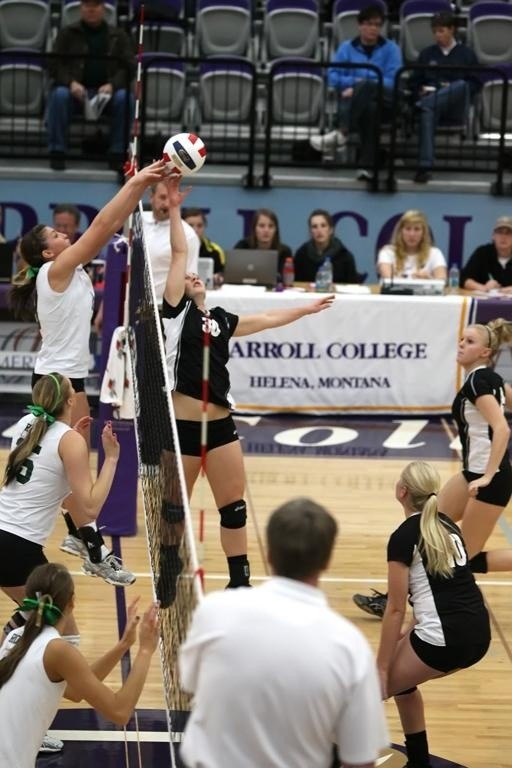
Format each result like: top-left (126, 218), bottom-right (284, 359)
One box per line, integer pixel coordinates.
top-left (0, 0), bottom-right (512, 171)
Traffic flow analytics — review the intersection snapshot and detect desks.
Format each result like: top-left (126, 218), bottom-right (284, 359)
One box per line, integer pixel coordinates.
top-left (0, 278), bottom-right (512, 419)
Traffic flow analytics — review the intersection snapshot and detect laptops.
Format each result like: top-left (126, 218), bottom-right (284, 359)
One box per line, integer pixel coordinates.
top-left (225, 249), bottom-right (277, 284)
top-left (198, 257), bottom-right (214, 292)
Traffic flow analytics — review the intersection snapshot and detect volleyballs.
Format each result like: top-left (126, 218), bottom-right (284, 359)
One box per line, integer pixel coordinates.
top-left (163, 131), bottom-right (205, 174)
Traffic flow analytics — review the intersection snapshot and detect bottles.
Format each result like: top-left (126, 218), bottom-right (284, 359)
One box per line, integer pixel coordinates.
top-left (283, 257), bottom-right (295, 286)
top-left (448, 263), bottom-right (460, 292)
top-left (315, 257), bottom-right (333, 291)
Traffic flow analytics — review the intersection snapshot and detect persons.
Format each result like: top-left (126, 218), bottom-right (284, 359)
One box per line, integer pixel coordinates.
top-left (21, 157), bottom-right (158, 583)
top-left (155, 171), bottom-right (334, 610)
top-left (144, 174), bottom-right (200, 314)
top-left (293, 209), bottom-right (357, 284)
top-left (376, 460), bottom-right (490, 766)
top-left (234, 208), bottom-right (293, 283)
top-left (52, 204), bottom-right (83, 246)
top-left (407, 9), bottom-right (479, 183)
top-left (376, 210), bottom-right (449, 287)
top-left (0, 373), bottom-right (119, 752)
top-left (352, 319), bottom-right (512, 618)
top-left (2, 564), bottom-right (164, 767)
top-left (309, 1), bottom-right (403, 181)
top-left (175, 495), bottom-right (391, 768)
top-left (459, 216), bottom-right (512, 294)
top-left (182, 208), bottom-right (226, 284)
top-left (46, 1), bottom-right (137, 171)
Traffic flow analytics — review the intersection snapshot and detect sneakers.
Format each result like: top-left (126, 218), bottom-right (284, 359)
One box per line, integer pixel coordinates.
top-left (80, 555), bottom-right (136, 586)
top-left (355, 168), bottom-right (373, 180)
top-left (58, 535), bottom-right (91, 560)
top-left (352, 588), bottom-right (388, 617)
top-left (310, 130), bottom-right (349, 154)
top-left (40, 735), bottom-right (65, 752)
top-left (155, 555), bottom-right (184, 609)
